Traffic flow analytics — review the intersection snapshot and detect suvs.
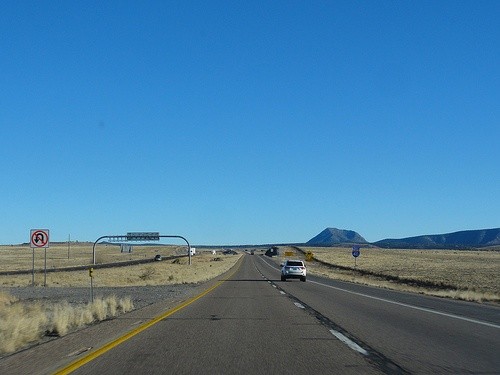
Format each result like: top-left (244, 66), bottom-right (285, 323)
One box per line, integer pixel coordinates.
top-left (279, 260), bottom-right (307, 282)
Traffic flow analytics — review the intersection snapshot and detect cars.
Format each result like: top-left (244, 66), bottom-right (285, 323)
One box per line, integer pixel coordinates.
top-left (154, 254), bottom-right (162, 261)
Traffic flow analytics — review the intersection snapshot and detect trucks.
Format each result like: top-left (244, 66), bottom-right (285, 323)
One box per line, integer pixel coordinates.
top-left (188, 248), bottom-right (196, 256)
